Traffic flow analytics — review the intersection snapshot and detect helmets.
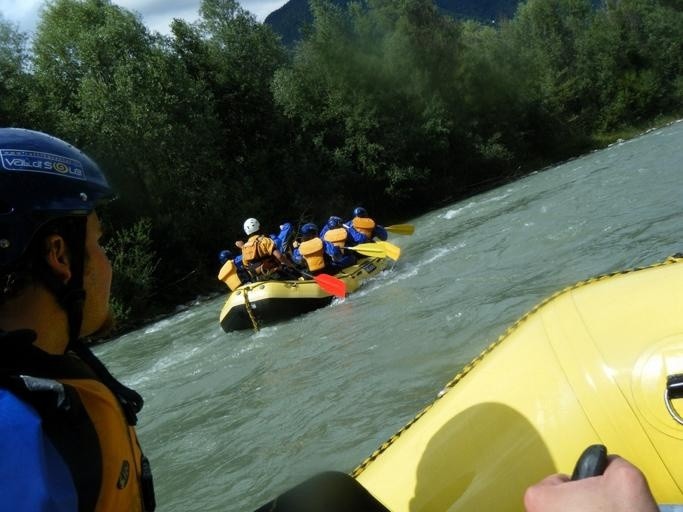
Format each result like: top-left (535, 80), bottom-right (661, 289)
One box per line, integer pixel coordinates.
top-left (0, 126), bottom-right (115, 283)
top-left (218, 206), bottom-right (368, 266)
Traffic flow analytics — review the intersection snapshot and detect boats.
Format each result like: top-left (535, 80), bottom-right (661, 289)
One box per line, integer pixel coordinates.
top-left (347, 253), bottom-right (683, 512)
top-left (219, 257), bottom-right (387, 333)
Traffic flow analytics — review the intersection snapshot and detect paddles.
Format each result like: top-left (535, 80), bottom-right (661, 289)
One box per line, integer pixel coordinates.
top-left (340, 223), bottom-right (416, 261)
top-left (284, 260), bottom-right (346, 298)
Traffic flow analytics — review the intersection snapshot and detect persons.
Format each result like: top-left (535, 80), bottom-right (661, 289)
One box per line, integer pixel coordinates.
top-left (0, 120), bottom-right (399, 512)
top-left (292, 221), bottom-right (352, 280)
top-left (241, 217), bottom-right (299, 281)
top-left (520, 450), bottom-right (661, 512)
top-left (218, 240), bottom-right (253, 291)
top-left (345, 205), bottom-right (388, 245)
top-left (267, 222), bottom-right (293, 254)
top-left (319, 215), bottom-right (370, 270)
